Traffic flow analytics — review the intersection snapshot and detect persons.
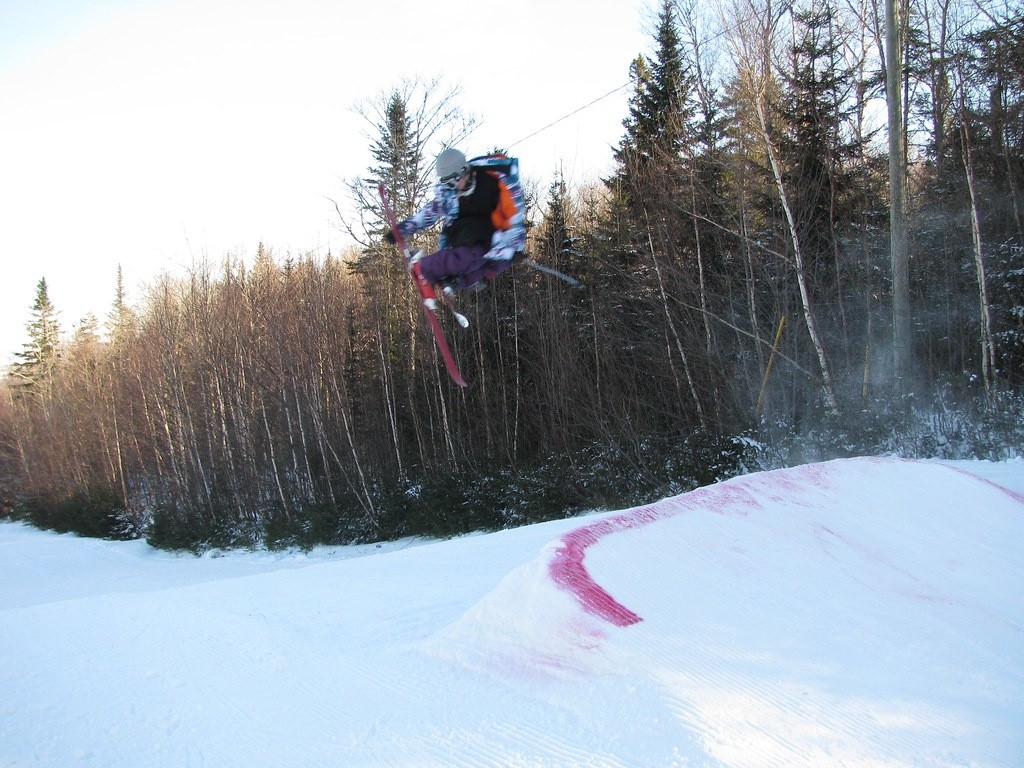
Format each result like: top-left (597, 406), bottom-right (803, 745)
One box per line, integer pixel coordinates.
top-left (383, 147), bottom-right (523, 303)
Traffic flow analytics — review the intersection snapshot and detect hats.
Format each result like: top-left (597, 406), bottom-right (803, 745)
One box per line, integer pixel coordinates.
top-left (436, 149), bottom-right (467, 178)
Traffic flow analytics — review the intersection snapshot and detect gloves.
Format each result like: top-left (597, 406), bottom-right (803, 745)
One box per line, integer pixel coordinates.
top-left (386, 228), bottom-right (397, 246)
top-left (512, 252), bottom-right (527, 266)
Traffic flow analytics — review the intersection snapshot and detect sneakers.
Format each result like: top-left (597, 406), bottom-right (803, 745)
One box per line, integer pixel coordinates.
top-left (410, 254), bottom-right (437, 310)
top-left (438, 277), bottom-right (454, 296)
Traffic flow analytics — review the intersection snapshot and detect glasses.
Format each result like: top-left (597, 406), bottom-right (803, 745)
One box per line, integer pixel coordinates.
top-left (440, 165), bottom-right (469, 184)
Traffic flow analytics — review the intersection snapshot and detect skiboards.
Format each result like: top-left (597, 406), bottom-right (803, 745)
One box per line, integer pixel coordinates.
top-left (378, 182), bottom-right (470, 390)
